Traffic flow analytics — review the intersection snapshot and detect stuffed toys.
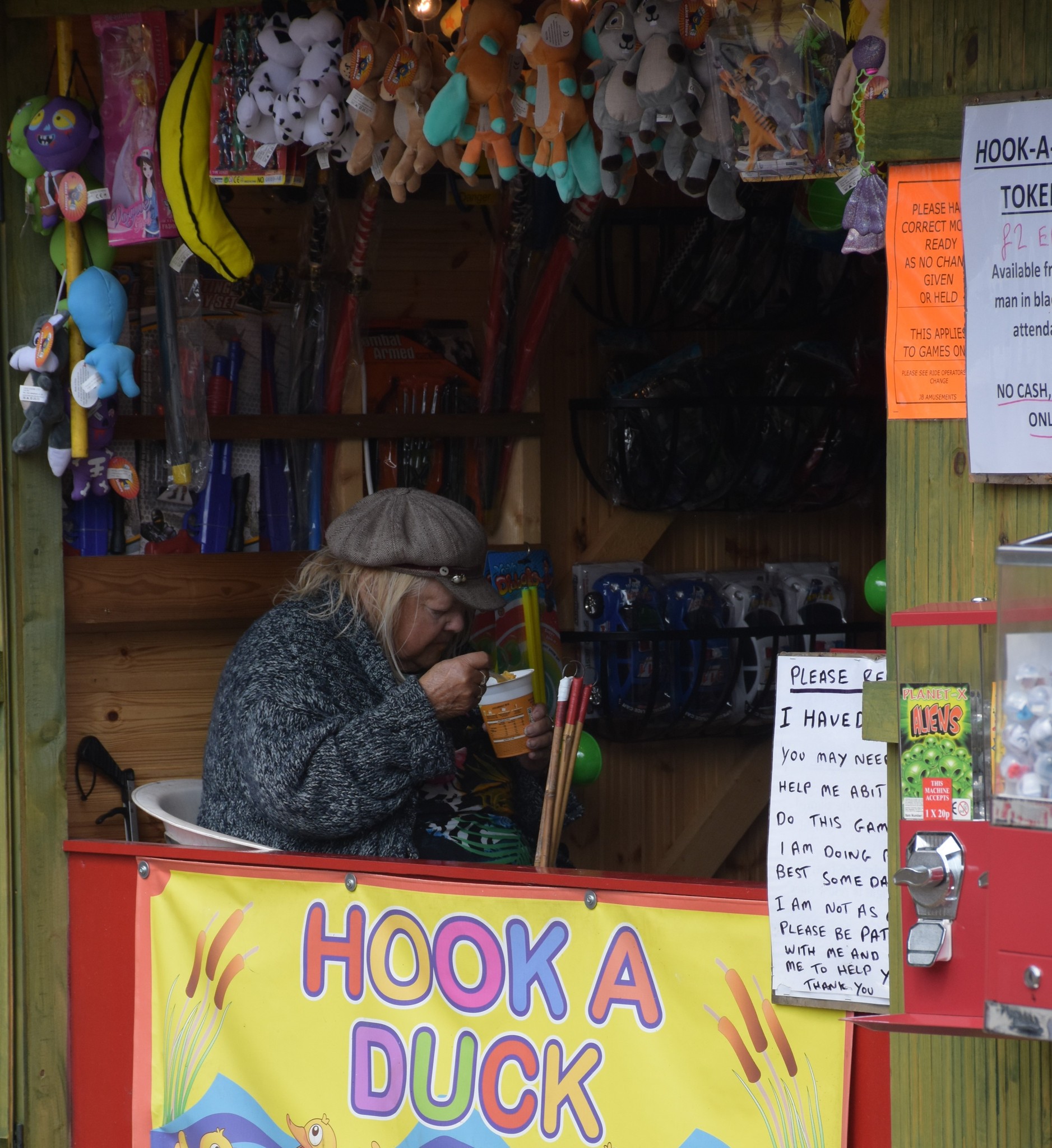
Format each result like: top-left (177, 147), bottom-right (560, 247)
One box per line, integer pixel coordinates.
top-left (65, 385), bottom-right (118, 501)
top-left (7, 309), bottom-right (72, 477)
top-left (6, 95), bottom-right (118, 329)
top-left (234, 0), bottom-right (747, 221)
top-left (67, 266), bottom-right (141, 399)
top-left (155, 39), bottom-right (257, 284)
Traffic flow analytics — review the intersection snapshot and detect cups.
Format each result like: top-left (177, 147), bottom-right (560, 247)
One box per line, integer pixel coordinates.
top-left (478, 669), bottom-right (538, 758)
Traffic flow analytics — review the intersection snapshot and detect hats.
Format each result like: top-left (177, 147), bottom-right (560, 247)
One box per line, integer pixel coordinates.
top-left (324, 483), bottom-right (508, 612)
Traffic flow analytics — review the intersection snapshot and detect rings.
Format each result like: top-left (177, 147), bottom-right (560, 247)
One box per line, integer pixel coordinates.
top-left (548, 716), bottom-right (554, 730)
top-left (477, 686), bottom-right (483, 699)
top-left (479, 670), bottom-right (487, 687)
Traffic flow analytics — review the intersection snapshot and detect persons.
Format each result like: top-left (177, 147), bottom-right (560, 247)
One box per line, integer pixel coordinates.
top-left (196, 488), bottom-right (584, 869)
